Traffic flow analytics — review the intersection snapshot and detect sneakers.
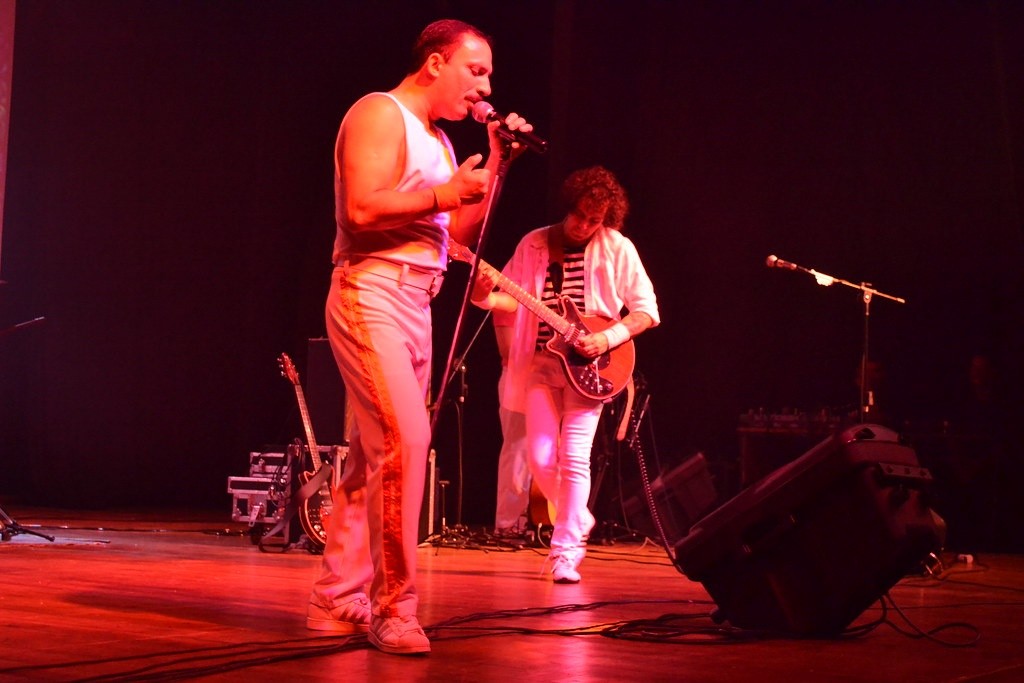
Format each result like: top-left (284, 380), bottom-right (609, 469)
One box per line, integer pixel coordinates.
top-left (367, 614), bottom-right (432, 653)
top-left (575, 508), bottom-right (595, 568)
top-left (306, 596), bottom-right (372, 632)
top-left (539, 552), bottom-right (581, 583)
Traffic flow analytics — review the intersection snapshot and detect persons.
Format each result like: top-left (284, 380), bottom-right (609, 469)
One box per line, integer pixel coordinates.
top-left (970, 352), bottom-right (997, 399)
top-left (308, 19), bottom-right (533, 654)
top-left (470, 166), bottom-right (661, 582)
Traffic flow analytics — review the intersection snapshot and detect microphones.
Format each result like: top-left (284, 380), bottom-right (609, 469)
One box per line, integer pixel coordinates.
top-left (471, 101), bottom-right (549, 155)
top-left (765, 255), bottom-right (798, 271)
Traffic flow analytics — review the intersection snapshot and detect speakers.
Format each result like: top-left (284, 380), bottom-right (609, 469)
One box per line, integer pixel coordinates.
top-left (622, 453), bottom-right (718, 545)
top-left (675, 424), bottom-right (950, 642)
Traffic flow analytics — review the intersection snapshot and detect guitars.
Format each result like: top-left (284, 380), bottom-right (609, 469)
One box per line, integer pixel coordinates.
top-left (448, 237), bottom-right (637, 402)
top-left (276, 347), bottom-right (336, 549)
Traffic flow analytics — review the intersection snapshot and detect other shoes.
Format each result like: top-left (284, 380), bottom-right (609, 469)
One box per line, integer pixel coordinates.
top-left (495, 529), bottom-right (534, 544)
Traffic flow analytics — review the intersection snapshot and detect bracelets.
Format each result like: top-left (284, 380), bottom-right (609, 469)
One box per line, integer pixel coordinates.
top-left (431, 184), bottom-right (461, 212)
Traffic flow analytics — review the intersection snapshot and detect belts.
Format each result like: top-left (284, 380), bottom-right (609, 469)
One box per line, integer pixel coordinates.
top-left (335, 254), bottom-right (444, 297)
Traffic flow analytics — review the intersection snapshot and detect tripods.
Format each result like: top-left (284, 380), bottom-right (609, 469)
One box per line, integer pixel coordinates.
top-left (422, 309), bottom-right (524, 553)
